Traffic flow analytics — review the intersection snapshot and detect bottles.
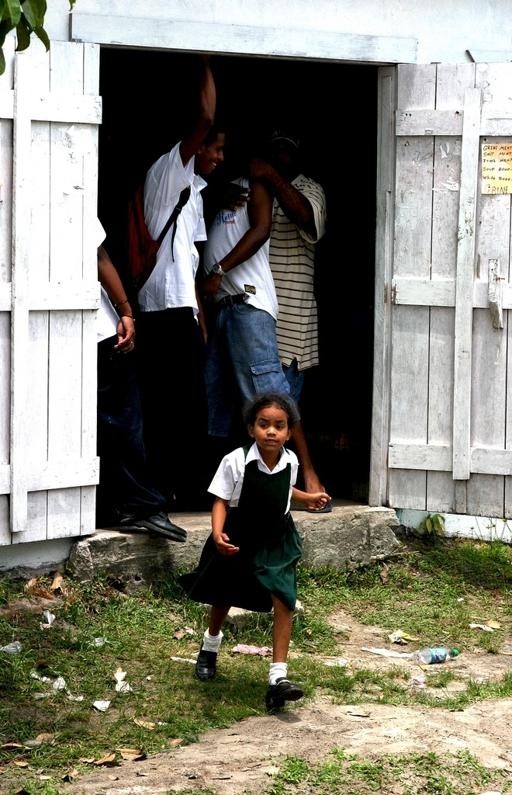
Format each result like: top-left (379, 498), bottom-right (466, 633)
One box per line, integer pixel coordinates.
top-left (420, 648), bottom-right (459, 664)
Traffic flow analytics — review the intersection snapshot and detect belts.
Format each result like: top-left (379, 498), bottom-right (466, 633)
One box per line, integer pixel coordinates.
top-left (209, 294), bottom-right (245, 307)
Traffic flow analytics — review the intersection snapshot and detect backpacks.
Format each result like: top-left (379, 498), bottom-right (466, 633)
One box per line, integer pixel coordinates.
top-left (109, 180), bottom-right (159, 299)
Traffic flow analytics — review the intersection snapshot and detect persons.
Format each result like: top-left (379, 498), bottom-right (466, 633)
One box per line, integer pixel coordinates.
top-left (172, 392), bottom-right (332, 700)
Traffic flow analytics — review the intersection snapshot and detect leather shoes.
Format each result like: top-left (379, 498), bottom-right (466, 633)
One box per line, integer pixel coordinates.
top-left (195, 640), bottom-right (217, 681)
top-left (123, 509), bottom-right (187, 543)
top-left (266, 678), bottom-right (302, 712)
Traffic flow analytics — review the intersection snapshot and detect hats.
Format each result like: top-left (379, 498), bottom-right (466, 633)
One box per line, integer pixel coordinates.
top-left (268, 127), bottom-right (301, 146)
